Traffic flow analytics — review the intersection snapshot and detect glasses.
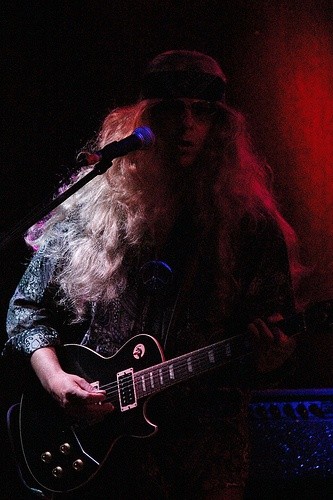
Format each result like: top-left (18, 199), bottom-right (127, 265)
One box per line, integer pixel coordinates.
top-left (150, 99), bottom-right (219, 126)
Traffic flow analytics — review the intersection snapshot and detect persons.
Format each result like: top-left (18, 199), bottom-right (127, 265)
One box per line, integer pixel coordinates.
top-left (6, 50), bottom-right (308, 499)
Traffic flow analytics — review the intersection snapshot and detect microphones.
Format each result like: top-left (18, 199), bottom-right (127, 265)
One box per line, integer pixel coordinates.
top-left (80, 127), bottom-right (154, 168)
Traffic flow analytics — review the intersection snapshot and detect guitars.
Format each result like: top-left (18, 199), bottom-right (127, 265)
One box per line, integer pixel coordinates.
top-left (18, 300), bottom-right (333, 494)
